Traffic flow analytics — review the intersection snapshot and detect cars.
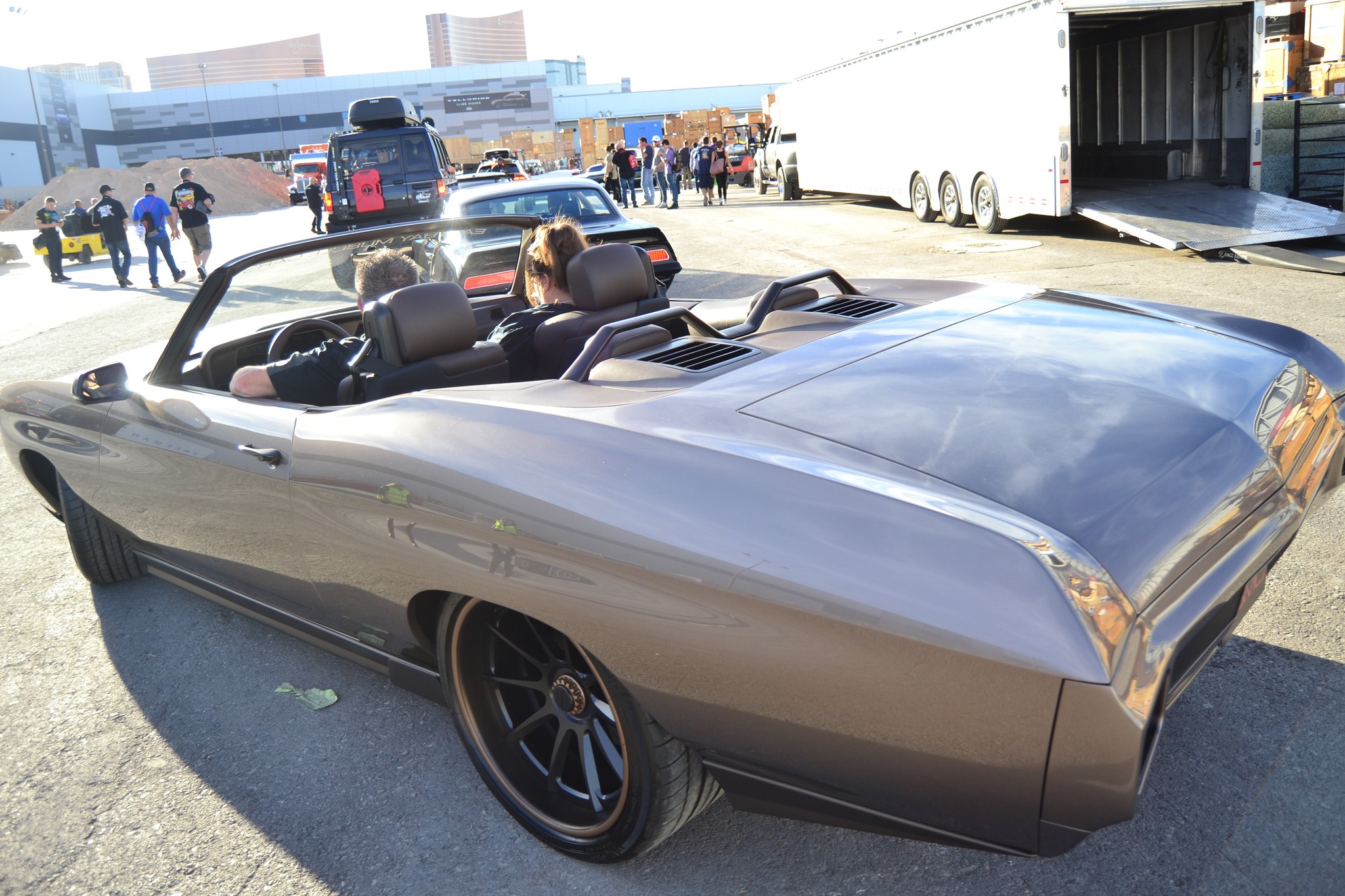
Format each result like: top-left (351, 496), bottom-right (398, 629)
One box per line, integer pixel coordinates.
top-left (0, 240), bottom-right (25, 266)
top-left (409, 176), bottom-right (682, 295)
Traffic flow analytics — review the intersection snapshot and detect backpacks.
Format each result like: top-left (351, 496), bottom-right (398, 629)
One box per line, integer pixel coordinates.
top-left (668, 147), bottom-right (683, 173)
top-left (140, 197), bottom-right (164, 238)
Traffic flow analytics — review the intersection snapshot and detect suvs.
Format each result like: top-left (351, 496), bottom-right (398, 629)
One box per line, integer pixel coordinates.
top-left (326, 97), bottom-right (459, 291)
top-left (458, 147), bottom-right (546, 177)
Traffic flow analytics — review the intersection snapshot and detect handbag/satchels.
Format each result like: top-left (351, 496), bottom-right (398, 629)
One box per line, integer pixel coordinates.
top-left (33, 234), bottom-right (48, 249)
top-left (628, 154), bottom-right (638, 168)
top-left (710, 151), bottom-right (724, 174)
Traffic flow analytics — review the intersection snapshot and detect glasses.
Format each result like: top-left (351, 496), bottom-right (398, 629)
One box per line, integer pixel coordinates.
top-left (653, 141), bottom-right (657, 142)
top-left (192, 175), bottom-right (194, 177)
top-left (313, 179), bottom-right (317, 181)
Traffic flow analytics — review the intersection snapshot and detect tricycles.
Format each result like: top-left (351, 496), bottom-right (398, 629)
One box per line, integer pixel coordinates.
top-left (34, 210), bottom-right (111, 268)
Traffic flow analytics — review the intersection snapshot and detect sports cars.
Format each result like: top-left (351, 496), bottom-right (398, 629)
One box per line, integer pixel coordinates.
top-left (0, 213), bottom-right (1344, 864)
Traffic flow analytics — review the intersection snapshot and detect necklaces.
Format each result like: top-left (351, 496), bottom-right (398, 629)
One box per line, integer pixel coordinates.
top-left (363, 151), bottom-right (380, 165)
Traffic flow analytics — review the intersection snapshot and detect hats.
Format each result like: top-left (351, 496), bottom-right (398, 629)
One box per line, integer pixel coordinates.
top-left (497, 156), bottom-right (504, 161)
top-left (135, 224), bottom-right (146, 241)
top-left (651, 135), bottom-right (660, 142)
top-left (145, 183), bottom-right (155, 191)
top-left (99, 185), bottom-right (115, 193)
top-left (179, 167), bottom-right (195, 174)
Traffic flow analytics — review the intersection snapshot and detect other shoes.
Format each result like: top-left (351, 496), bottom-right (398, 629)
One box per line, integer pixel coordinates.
top-left (703, 195), bottom-right (727, 206)
top-left (117, 274), bottom-right (133, 288)
top-left (684, 187), bottom-right (693, 190)
top-left (322, 209), bottom-right (326, 212)
top-left (622, 204), bottom-right (638, 208)
top-left (641, 201), bottom-right (655, 205)
top-left (311, 228), bottom-right (326, 235)
top-left (656, 202), bottom-right (679, 210)
top-left (52, 275), bottom-right (72, 282)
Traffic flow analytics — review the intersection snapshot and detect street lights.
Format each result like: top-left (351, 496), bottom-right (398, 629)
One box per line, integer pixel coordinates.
top-left (9, 5), bottom-right (53, 181)
top-left (273, 81), bottom-right (291, 177)
top-left (198, 63), bottom-right (218, 158)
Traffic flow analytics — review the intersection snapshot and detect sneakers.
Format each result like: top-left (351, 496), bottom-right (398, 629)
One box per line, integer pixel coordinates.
top-left (197, 265), bottom-right (207, 282)
top-left (152, 283), bottom-right (159, 288)
top-left (174, 270), bottom-right (185, 282)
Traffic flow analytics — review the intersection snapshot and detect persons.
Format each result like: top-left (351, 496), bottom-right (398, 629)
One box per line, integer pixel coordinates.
top-left (92, 184), bottom-right (133, 289)
top-left (304, 177), bottom-right (327, 234)
top-left (35, 196), bottom-right (72, 283)
top-left (225, 244), bottom-right (422, 410)
top-left (69, 199), bottom-right (87, 216)
top-left (416, 141), bottom-right (456, 173)
top-left (87, 197), bottom-right (99, 216)
top-left (449, 126), bottom-right (771, 209)
top-left (485, 206), bottom-right (606, 381)
top-left (169, 166), bottom-right (213, 283)
top-left (386, 139), bottom-right (430, 171)
top-left (131, 182), bottom-right (185, 289)
top-left (320, 173), bottom-right (328, 213)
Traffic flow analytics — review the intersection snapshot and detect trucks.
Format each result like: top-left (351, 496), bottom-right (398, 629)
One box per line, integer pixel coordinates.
top-left (751, 0), bottom-right (1345, 251)
top-left (288, 152), bottom-right (328, 206)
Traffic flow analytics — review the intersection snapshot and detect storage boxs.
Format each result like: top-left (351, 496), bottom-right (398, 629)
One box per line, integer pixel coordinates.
top-left (347, 96), bottom-right (420, 130)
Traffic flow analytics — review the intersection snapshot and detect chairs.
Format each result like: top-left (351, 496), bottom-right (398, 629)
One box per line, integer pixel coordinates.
top-left (554, 199), bottom-right (582, 217)
top-left (532, 242), bottom-right (689, 382)
top-left (336, 281), bottom-right (508, 403)
